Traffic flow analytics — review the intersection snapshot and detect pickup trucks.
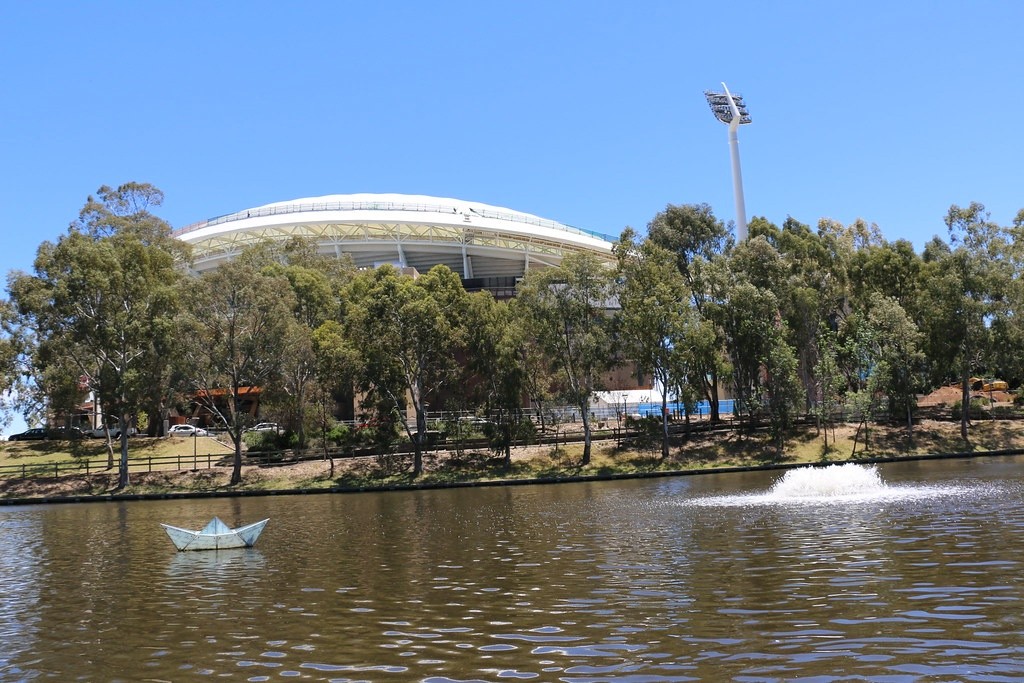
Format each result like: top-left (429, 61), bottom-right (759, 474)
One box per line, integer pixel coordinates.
top-left (84, 425), bottom-right (137, 438)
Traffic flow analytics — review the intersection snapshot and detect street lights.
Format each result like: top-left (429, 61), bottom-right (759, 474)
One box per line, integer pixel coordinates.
top-left (703, 82), bottom-right (752, 244)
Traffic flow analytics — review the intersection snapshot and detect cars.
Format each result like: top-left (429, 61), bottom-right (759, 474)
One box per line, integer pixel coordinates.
top-left (246, 422), bottom-right (283, 432)
top-left (48, 427), bottom-right (84, 440)
top-left (169, 425), bottom-right (205, 437)
top-left (8, 428), bottom-right (47, 441)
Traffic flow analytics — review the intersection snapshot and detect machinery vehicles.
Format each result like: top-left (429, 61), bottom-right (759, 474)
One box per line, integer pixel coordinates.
top-left (948, 376), bottom-right (1012, 403)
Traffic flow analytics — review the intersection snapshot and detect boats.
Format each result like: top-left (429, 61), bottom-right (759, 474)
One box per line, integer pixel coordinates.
top-left (159, 516), bottom-right (270, 552)
